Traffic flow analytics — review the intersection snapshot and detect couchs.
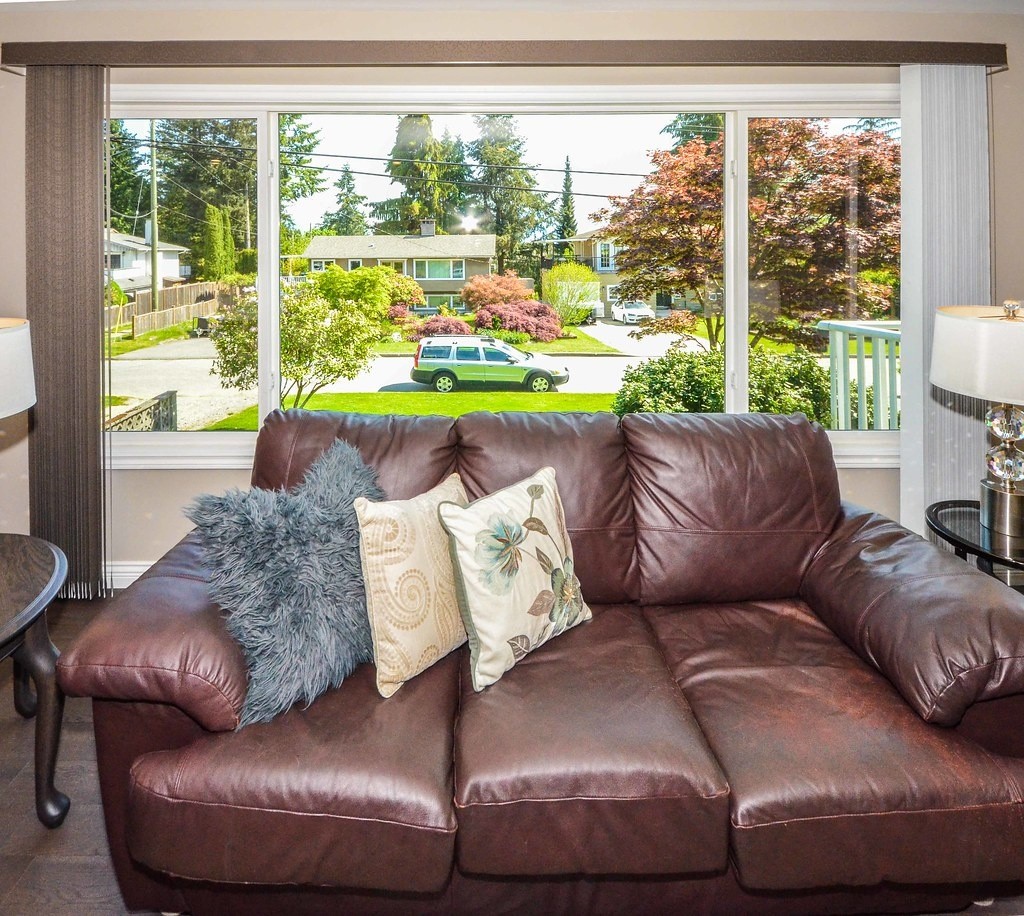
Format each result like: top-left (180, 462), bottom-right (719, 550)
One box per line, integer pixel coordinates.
top-left (61, 413), bottom-right (1023, 916)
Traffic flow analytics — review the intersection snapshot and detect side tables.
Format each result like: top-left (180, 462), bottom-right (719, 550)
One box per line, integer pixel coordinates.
top-left (924, 500), bottom-right (1024, 592)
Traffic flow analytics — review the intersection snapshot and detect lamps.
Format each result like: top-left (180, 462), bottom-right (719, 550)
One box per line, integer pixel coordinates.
top-left (0, 314), bottom-right (37, 423)
top-left (931, 300), bottom-right (1024, 538)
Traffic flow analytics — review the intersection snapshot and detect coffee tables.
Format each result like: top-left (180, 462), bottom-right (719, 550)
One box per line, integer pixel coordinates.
top-left (0, 532), bottom-right (70, 825)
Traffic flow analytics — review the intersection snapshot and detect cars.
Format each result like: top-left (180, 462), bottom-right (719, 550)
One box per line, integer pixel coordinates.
top-left (611, 299), bottom-right (656, 325)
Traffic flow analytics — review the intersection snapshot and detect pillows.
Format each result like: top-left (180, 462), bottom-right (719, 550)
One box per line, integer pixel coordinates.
top-left (440, 467), bottom-right (594, 694)
top-left (181, 436), bottom-right (387, 730)
top-left (353, 470), bottom-right (467, 697)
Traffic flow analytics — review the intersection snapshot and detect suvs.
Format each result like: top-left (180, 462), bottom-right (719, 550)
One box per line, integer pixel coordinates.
top-left (410, 334), bottom-right (570, 394)
